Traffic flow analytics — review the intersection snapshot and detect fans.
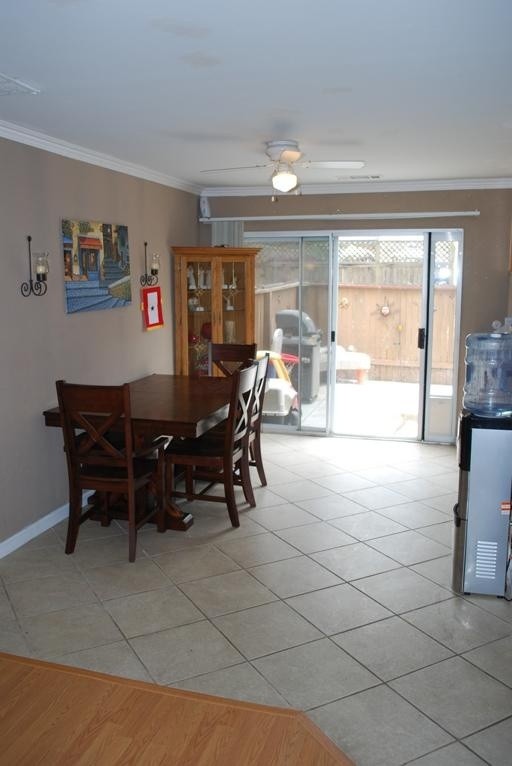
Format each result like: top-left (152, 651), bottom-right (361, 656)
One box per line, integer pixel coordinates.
top-left (198, 138), bottom-right (367, 202)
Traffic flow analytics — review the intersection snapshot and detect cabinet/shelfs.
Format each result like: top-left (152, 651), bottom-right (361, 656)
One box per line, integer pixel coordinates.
top-left (172, 247), bottom-right (260, 379)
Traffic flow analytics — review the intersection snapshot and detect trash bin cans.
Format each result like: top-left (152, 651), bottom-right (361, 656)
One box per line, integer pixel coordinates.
top-left (276, 310), bottom-right (320, 404)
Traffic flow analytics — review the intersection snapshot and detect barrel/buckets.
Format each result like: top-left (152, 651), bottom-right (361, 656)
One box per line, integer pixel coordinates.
top-left (462, 333), bottom-right (512, 418)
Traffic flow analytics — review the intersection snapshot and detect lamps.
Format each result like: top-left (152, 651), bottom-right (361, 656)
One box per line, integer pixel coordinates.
top-left (270, 162), bottom-right (300, 195)
top-left (19, 236), bottom-right (51, 299)
top-left (137, 241), bottom-right (163, 287)
